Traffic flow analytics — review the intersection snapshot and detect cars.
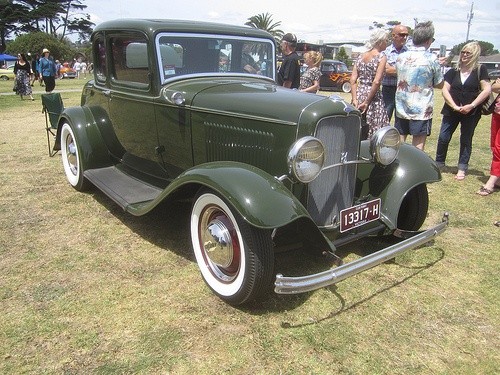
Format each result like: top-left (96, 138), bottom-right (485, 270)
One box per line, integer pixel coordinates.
top-left (299, 59), bottom-right (356, 94)
top-left (59, 68), bottom-right (77, 79)
top-left (53, 17), bottom-right (451, 308)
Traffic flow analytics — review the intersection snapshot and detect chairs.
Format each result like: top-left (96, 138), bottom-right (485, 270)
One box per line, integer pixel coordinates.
top-left (41, 93), bottom-right (67, 157)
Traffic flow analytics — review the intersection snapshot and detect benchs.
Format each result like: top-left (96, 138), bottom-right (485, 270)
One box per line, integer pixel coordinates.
top-left (114, 65), bottom-right (185, 86)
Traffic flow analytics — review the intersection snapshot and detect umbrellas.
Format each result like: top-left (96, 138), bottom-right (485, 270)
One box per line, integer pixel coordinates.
top-left (0, 53), bottom-right (18, 64)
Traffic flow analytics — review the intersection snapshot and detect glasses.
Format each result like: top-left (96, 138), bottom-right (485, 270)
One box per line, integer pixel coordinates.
top-left (429, 37), bottom-right (435, 43)
top-left (395, 33), bottom-right (409, 37)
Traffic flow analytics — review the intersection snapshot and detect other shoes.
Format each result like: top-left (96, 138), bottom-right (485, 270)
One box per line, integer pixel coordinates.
top-left (31, 98), bottom-right (35, 101)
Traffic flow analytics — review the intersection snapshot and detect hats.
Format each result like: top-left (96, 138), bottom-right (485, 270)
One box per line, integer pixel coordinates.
top-left (283, 33), bottom-right (297, 43)
top-left (42, 49), bottom-right (50, 52)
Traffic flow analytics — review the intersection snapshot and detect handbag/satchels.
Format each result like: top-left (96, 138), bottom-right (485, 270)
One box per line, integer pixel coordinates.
top-left (477, 65), bottom-right (496, 114)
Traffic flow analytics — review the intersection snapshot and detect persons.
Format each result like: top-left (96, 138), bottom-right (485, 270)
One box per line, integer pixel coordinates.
top-left (477, 75), bottom-right (500, 196)
top-left (350, 29), bottom-right (391, 140)
top-left (382, 25), bottom-right (409, 124)
top-left (494, 220), bottom-right (500, 227)
top-left (39, 49), bottom-right (56, 93)
top-left (298, 50), bottom-right (323, 94)
top-left (55, 58), bottom-right (93, 80)
top-left (435, 41), bottom-right (491, 179)
top-left (218, 43), bottom-right (273, 79)
top-left (13, 54), bottom-right (35, 101)
top-left (1, 64), bottom-right (6, 69)
top-left (27, 53), bottom-right (44, 87)
top-left (394, 21), bottom-right (445, 150)
top-left (277, 33), bottom-right (300, 90)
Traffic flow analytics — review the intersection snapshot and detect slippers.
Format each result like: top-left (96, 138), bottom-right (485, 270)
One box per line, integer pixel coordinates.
top-left (455, 173), bottom-right (464, 180)
top-left (478, 187), bottom-right (493, 196)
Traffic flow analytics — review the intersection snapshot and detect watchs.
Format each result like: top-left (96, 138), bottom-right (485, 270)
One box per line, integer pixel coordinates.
top-left (471, 103), bottom-right (475, 108)
